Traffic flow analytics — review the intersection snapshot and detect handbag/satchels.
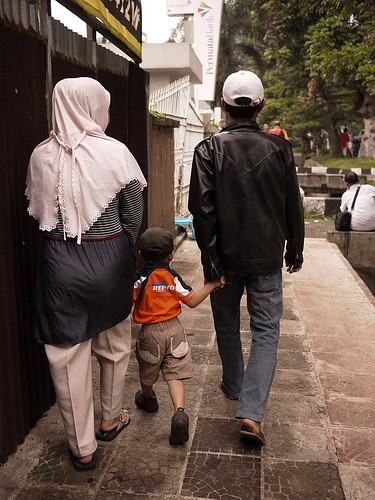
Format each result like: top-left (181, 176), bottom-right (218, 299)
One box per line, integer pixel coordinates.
top-left (335, 212), bottom-right (351, 231)
top-left (279, 128), bottom-right (284, 139)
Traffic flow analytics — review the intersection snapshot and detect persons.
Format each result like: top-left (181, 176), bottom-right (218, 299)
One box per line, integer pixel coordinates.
top-left (261, 123), bottom-right (364, 159)
top-left (340, 172), bottom-right (375, 232)
top-left (187, 71), bottom-right (305, 445)
top-left (133, 226), bottom-right (225, 446)
top-left (25, 78), bottom-right (149, 472)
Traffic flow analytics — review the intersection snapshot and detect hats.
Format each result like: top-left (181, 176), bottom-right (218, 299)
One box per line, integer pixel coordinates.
top-left (222, 70), bottom-right (264, 107)
top-left (139, 227), bottom-right (174, 257)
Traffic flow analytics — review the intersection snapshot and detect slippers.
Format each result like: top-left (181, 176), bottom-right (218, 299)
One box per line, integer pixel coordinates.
top-left (219, 378), bottom-right (239, 400)
top-left (240, 425), bottom-right (266, 445)
top-left (71, 450), bottom-right (96, 472)
top-left (96, 408), bottom-right (130, 442)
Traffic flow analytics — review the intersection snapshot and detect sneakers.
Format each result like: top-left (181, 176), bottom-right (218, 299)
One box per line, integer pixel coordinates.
top-left (169, 407), bottom-right (189, 443)
top-left (135, 389), bottom-right (159, 413)
